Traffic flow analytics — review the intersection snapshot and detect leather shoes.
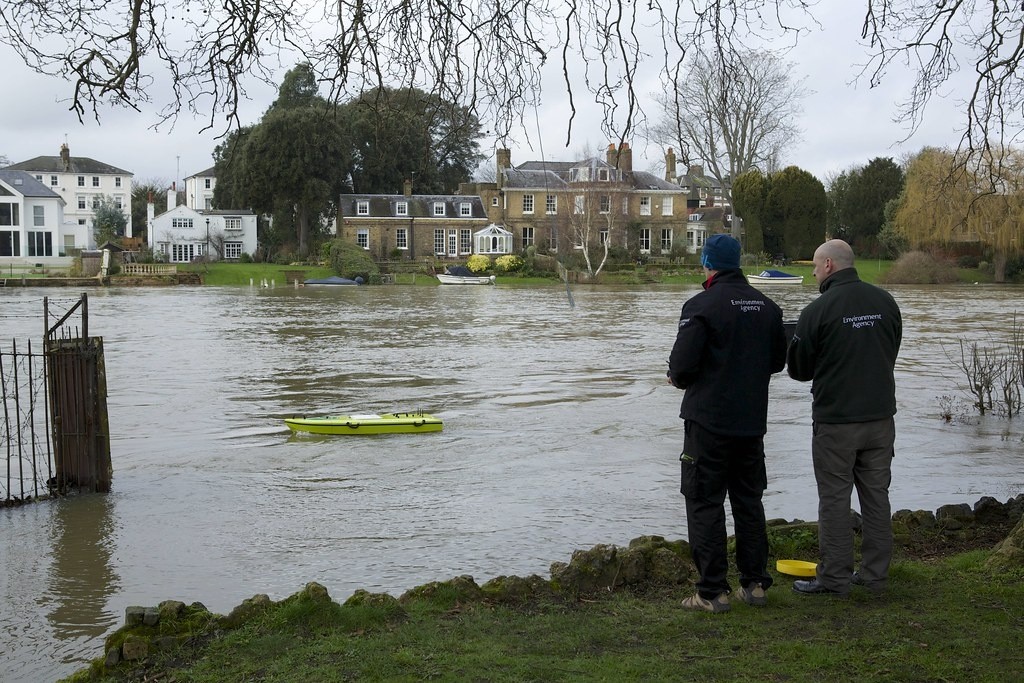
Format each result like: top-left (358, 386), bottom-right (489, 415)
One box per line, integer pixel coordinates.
top-left (792, 578), bottom-right (848, 596)
top-left (851, 570), bottom-right (866, 586)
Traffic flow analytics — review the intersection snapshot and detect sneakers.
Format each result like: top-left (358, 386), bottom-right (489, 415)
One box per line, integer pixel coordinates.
top-left (735, 582), bottom-right (770, 608)
top-left (682, 592), bottom-right (730, 614)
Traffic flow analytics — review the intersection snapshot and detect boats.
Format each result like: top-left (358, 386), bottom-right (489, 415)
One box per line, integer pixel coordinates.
top-left (437, 268), bottom-right (495, 285)
top-left (747, 270), bottom-right (805, 285)
top-left (283, 414), bottom-right (442, 434)
top-left (300, 275), bottom-right (362, 287)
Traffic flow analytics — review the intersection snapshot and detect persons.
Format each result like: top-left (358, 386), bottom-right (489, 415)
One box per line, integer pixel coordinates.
top-left (788, 239), bottom-right (902, 596)
top-left (667, 235), bottom-right (788, 613)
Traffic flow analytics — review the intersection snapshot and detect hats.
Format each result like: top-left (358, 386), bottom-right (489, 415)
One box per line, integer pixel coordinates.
top-left (701, 234), bottom-right (741, 271)
